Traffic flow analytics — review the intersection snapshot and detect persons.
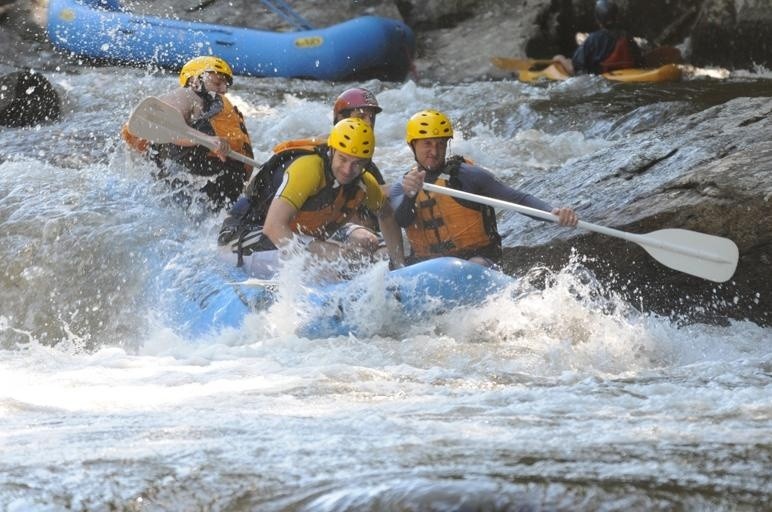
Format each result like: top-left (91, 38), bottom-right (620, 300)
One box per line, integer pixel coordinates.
top-left (525, 0), bottom-right (640, 74)
top-left (124, 56), bottom-right (254, 218)
top-left (216, 117), bottom-right (404, 282)
top-left (390, 110), bottom-right (578, 271)
top-left (271, 87), bottom-right (383, 232)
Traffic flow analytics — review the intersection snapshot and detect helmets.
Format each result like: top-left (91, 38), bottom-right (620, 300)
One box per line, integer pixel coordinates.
top-left (406, 110), bottom-right (453, 144)
top-left (327, 117), bottom-right (375, 159)
top-left (333, 88), bottom-right (383, 116)
top-left (595, 0), bottom-right (618, 21)
top-left (177, 55), bottom-right (233, 87)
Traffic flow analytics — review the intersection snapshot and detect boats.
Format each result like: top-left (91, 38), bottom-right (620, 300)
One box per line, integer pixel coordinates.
top-left (46, 0), bottom-right (414, 82)
top-left (518, 59), bottom-right (694, 88)
top-left (97, 177), bottom-right (536, 342)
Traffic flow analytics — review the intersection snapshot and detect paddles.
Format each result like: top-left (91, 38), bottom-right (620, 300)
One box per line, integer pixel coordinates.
top-left (127, 95), bottom-right (263, 170)
top-left (488, 56), bottom-right (559, 72)
top-left (411, 181), bottom-right (740, 283)
top-left (694, 68), bottom-right (772, 79)
